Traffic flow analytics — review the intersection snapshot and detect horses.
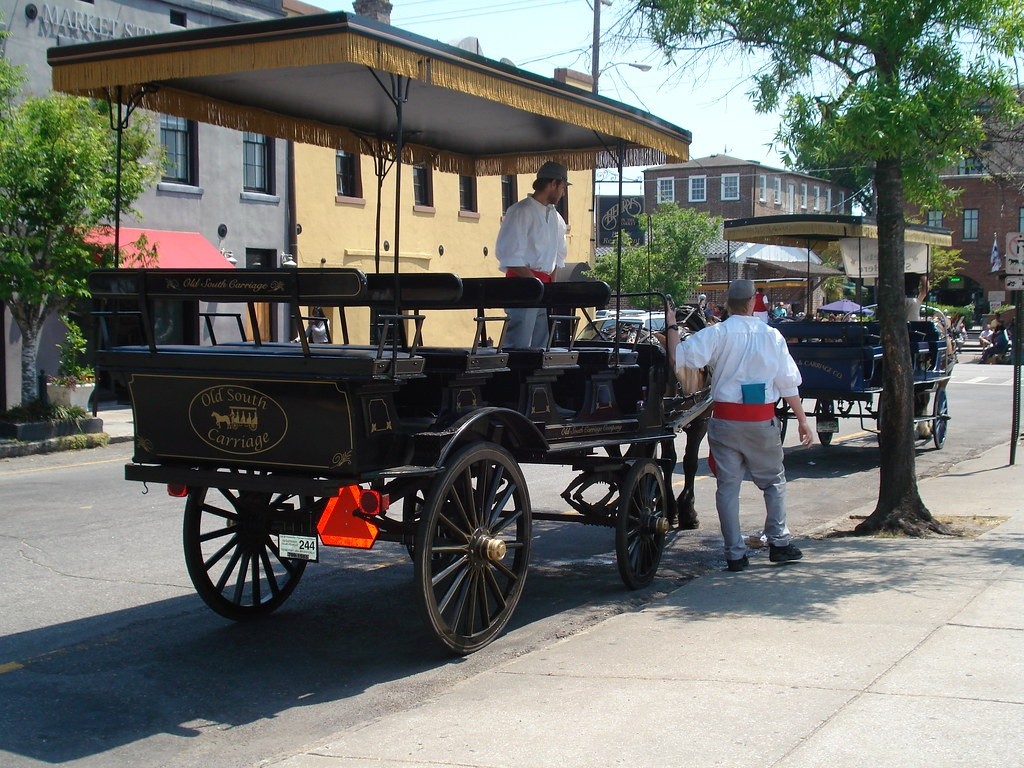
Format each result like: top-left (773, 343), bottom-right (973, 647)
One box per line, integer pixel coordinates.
top-left (913, 311), bottom-right (961, 439)
top-left (591, 303), bottom-right (712, 530)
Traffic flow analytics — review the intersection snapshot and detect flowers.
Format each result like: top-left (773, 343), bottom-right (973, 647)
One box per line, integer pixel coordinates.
top-left (45, 316), bottom-right (96, 392)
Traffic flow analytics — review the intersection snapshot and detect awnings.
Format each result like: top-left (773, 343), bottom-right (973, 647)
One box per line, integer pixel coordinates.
top-left (67, 224), bottom-right (234, 269)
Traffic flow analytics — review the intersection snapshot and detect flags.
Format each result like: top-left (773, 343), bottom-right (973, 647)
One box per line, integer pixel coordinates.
top-left (991, 238), bottom-right (1001, 272)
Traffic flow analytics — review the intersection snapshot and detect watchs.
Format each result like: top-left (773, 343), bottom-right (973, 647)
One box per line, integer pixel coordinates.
top-left (668, 324), bottom-right (679, 331)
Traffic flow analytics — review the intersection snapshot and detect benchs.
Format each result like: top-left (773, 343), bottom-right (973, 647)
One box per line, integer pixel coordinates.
top-left (88, 269), bottom-right (641, 471)
top-left (767, 322), bottom-right (947, 389)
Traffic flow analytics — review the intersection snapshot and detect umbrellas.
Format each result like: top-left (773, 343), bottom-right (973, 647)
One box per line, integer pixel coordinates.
top-left (816, 299), bottom-right (874, 321)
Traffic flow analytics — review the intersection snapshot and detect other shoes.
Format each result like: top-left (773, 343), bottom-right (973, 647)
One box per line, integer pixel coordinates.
top-left (554, 406), bottom-right (578, 419)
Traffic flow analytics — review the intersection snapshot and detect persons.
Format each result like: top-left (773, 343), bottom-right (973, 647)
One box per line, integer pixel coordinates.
top-left (667, 279), bottom-right (814, 573)
top-left (495, 161), bottom-right (573, 348)
top-left (290, 306), bottom-right (331, 344)
top-left (703, 272), bottom-right (1016, 375)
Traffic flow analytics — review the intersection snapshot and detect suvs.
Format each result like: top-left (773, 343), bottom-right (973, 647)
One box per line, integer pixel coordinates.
top-left (575, 306), bottom-right (720, 354)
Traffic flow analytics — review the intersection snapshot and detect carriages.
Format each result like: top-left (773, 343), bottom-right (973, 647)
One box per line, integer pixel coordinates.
top-left (722, 210), bottom-right (961, 450)
top-left (49, 0), bottom-right (730, 657)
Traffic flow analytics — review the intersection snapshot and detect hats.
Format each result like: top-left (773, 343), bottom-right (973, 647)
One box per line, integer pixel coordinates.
top-left (728, 279), bottom-right (757, 300)
top-left (537, 162), bottom-right (572, 187)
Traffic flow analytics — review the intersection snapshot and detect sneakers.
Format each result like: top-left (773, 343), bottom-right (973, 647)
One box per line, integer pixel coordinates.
top-left (769, 542), bottom-right (803, 562)
top-left (726, 554), bottom-right (748, 572)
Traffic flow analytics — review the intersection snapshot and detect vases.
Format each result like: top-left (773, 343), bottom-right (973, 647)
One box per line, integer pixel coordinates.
top-left (45, 382), bottom-right (95, 414)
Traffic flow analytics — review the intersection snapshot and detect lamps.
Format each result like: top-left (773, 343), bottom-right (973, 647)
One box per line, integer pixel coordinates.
top-left (281, 252), bottom-right (297, 268)
top-left (220, 248), bottom-right (238, 267)
